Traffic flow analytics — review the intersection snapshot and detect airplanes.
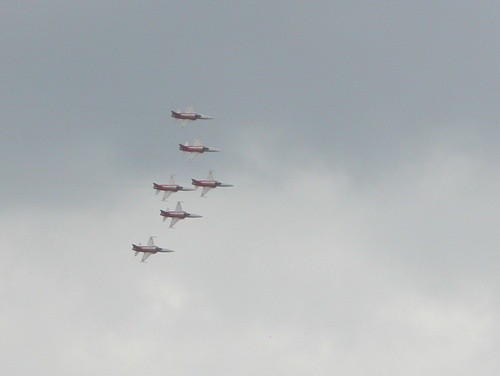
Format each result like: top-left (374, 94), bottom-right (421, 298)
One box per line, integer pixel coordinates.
top-left (179, 143), bottom-right (222, 159)
top-left (191, 170), bottom-right (232, 198)
top-left (170, 110), bottom-right (214, 122)
top-left (160, 203), bottom-right (203, 229)
top-left (132, 237), bottom-right (174, 263)
top-left (153, 177), bottom-right (194, 200)
